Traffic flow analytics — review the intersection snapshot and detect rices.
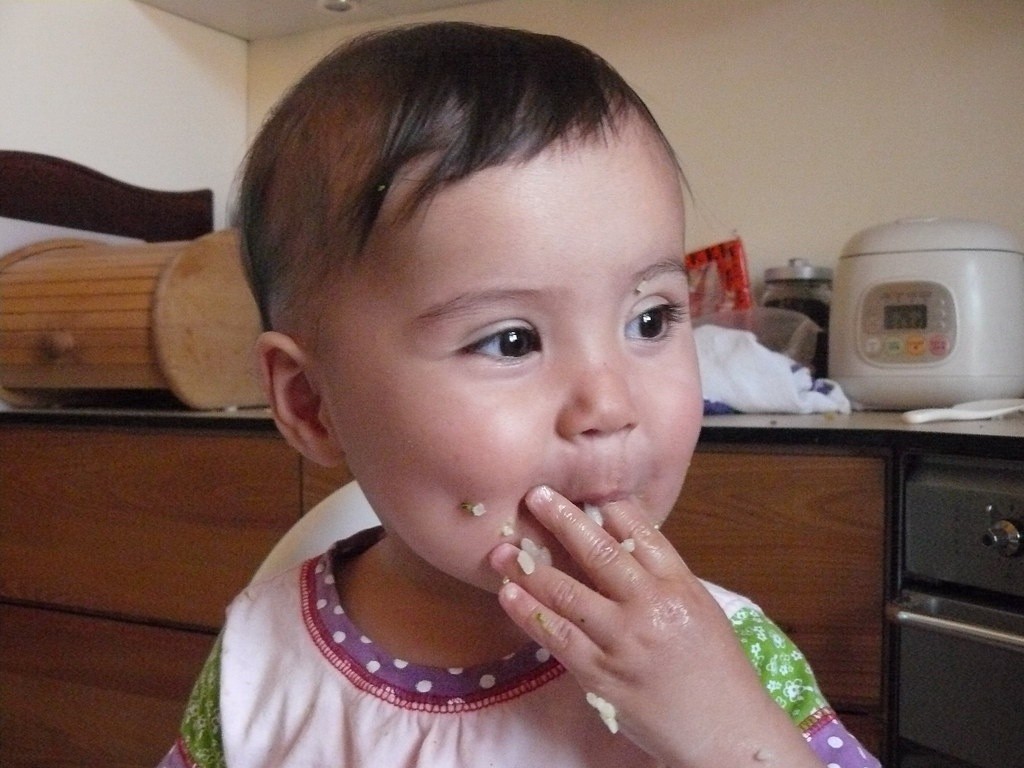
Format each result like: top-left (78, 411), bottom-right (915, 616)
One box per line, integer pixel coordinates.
top-left (756, 750), bottom-right (769, 761)
top-left (467, 500), bottom-right (636, 734)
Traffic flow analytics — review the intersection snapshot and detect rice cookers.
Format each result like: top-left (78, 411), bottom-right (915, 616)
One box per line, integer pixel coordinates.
top-left (827, 218), bottom-right (1024, 412)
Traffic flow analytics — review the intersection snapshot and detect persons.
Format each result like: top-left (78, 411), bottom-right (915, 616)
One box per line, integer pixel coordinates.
top-left (163, 21), bottom-right (881, 768)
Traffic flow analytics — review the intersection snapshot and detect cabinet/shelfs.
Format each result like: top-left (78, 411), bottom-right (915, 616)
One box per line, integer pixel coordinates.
top-left (0, 408), bottom-right (1024, 768)
